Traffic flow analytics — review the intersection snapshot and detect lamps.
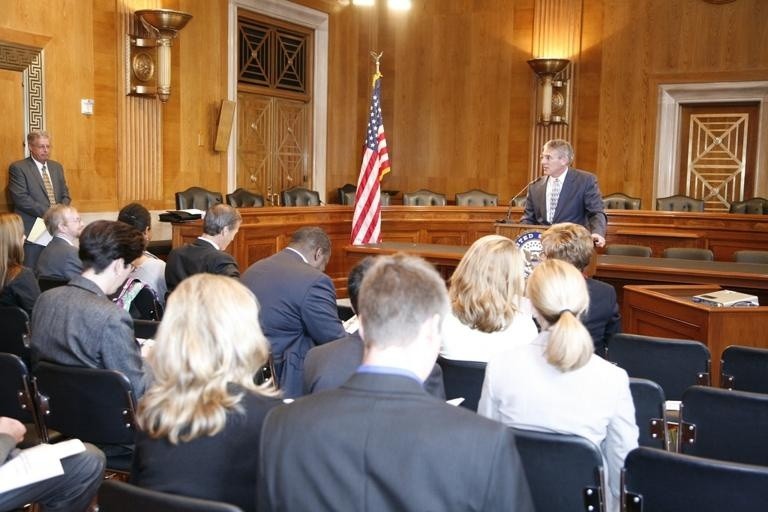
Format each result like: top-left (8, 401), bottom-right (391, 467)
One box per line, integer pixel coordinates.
top-left (527, 57), bottom-right (570, 127)
top-left (126, 9), bottom-right (193, 102)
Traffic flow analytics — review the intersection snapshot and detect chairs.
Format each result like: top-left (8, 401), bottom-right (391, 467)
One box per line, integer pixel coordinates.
top-left (0, 184), bottom-right (768, 511)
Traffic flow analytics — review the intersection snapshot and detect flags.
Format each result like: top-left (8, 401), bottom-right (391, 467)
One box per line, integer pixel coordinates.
top-left (348, 72), bottom-right (392, 245)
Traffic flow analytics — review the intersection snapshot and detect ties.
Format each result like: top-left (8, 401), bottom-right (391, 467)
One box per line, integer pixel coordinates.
top-left (549, 179), bottom-right (560, 224)
top-left (42, 166), bottom-right (57, 209)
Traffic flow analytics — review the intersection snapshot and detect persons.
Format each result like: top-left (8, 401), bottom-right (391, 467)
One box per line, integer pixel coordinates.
top-left (239, 225), bottom-right (350, 400)
top-left (0, 415), bottom-right (107, 512)
top-left (439, 235), bottom-right (540, 364)
top-left (36, 205), bottom-right (85, 281)
top-left (254, 251), bottom-right (533, 511)
top-left (477, 258), bottom-right (641, 511)
top-left (9, 131), bottom-right (72, 269)
top-left (519, 138), bottom-right (607, 249)
top-left (542, 222), bottom-right (622, 357)
top-left (30, 220), bottom-right (155, 470)
top-left (165, 205), bottom-right (244, 290)
top-left (0, 213), bottom-right (42, 319)
top-left (117, 205), bottom-right (169, 316)
top-left (302, 255), bottom-right (446, 403)
top-left (130, 273), bottom-right (288, 512)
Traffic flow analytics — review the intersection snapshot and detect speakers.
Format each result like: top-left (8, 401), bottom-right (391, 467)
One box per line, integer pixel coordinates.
top-left (213, 97), bottom-right (237, 156)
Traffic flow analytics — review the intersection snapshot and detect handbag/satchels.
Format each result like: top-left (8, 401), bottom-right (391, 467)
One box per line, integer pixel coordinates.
top-left (159, 211), bottom-right (201, 223)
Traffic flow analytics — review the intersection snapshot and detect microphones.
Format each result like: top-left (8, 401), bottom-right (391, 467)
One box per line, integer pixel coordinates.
top-left (498, 176), bottom-right (543, 224)
